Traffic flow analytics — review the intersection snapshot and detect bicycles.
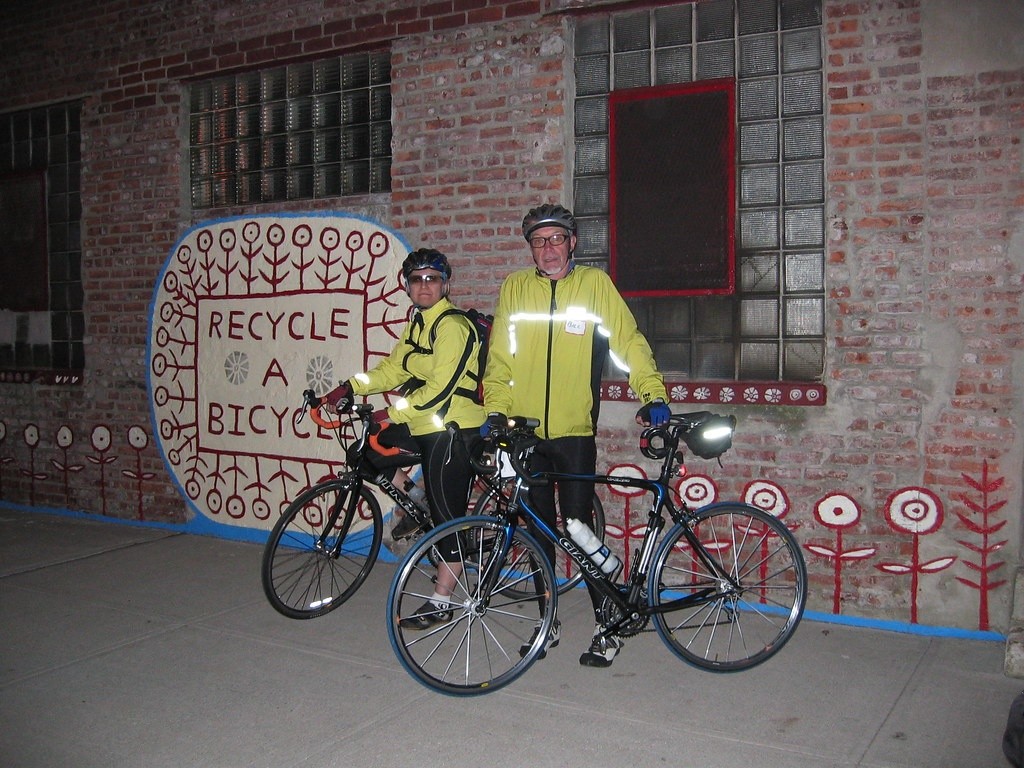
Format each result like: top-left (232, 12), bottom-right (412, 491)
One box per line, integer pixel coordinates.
top-left (260, 388), bottom-right (606, 620)
top-left (386, 410), bottom-right (808, 698)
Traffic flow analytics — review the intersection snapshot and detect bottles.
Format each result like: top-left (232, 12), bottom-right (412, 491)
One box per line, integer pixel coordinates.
top-left (403, 480), bottom-right (431, 517)
top-left (566, 518), bottom-right (619, 573)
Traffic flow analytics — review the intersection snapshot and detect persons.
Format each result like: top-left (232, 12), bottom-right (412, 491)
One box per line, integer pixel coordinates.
top-left (481, 203), bottom-right (672, 667)
top-left (322, 249), bottom-right (486, 630)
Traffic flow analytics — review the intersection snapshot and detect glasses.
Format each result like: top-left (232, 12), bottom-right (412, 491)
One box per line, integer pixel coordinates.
top-left (529, 234), bottom-right (569, 248)
top-left (408, 275), bottom-right (444, 284)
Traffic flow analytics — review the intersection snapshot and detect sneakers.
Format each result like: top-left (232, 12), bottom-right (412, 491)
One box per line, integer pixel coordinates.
top-left (400, 601), bottom-right (454, 630)
top-left (392, 507), bottom-right (431, 539)
top-left (580, 622), bottom-right (620, 667)
top-left (519, 619), bottom-right (562, 660)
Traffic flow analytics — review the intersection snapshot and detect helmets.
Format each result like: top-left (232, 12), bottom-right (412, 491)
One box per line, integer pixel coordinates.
top-left (521, 204), bottom-right (576, 242)
top-left (402, 248), bottom-right (452, 279)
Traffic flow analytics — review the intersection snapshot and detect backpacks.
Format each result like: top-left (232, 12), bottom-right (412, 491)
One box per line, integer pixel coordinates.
top-left (399, 309), bottom-right (494, 407)
